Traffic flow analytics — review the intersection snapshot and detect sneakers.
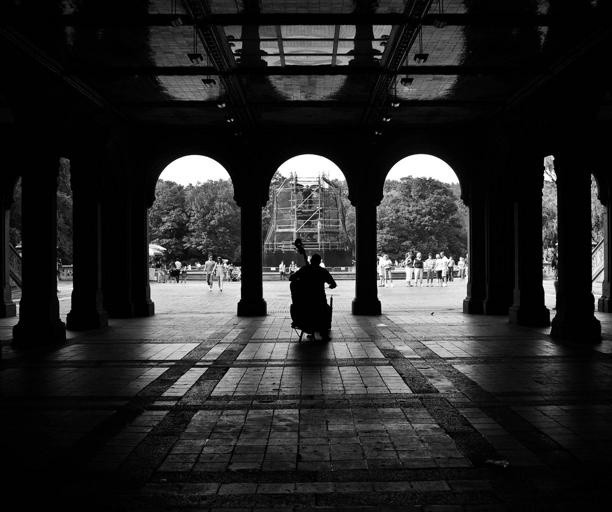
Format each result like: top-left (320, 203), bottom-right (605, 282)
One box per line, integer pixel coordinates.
top-left (306, 335), bottom-right (331, 342)
top-left (407, 283), bottom-right (447, 288)
top-left (380, 283), bottom-right (394, 289)
top-left (205, 285), bottom-right (224, 293)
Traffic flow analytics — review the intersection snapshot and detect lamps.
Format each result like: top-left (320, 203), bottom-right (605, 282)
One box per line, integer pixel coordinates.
top-left (390, 15), bottom-right (429, 109)
top-left (166, 0), bottom-right (217, 89)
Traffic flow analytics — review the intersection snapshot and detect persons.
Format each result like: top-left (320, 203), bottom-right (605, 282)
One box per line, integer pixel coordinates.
top-left (377, 252), bottom-right (393, 289)
top-left (155, 258), bottom-right (191, 284)
top-left (205, 255), bottom-right (233, 292)
top-left (288, 253), bottom-right (338, 341)
top-left (405, 251), bottom-right (465, 287)
top-left (279, 261), bottom-right (297, 282)
